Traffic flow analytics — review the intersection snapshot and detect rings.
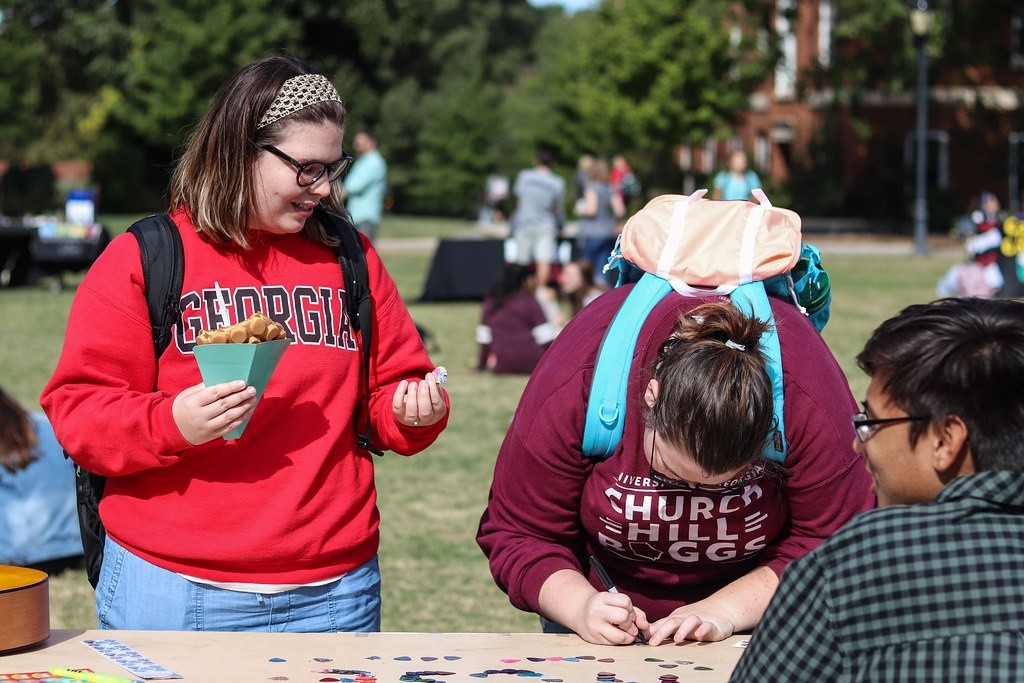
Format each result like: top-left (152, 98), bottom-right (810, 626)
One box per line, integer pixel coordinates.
top-left (408, 418), bottom-right (420, 425)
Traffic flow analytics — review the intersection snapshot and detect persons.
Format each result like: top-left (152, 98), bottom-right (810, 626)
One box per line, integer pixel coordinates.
top-left (0, 385), bottom-right (85, 576)
top-left (342, 126), bottom-right (387, 242)
top-left (475, 188), bottom-right (877, 646)
top-left (935, 192), bottom-right (1024, 299)
top-left (727, 295), bottom-right (1024, 683)
top-left (476, 141), bottom-right (766, 373)
top-left (39, 55), bottom-right (450, 633)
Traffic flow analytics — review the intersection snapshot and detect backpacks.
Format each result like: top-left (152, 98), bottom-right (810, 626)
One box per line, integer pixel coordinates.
top-left (581, 188), bottom-right (833, 462)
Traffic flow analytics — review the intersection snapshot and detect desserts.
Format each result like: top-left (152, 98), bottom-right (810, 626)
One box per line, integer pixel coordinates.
top-left (193, 313), bottom-right (290, 346)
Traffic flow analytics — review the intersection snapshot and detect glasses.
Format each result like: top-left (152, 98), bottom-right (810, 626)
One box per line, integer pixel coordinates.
top-left (261, 144), bottom-right (355, 186)
top-left (851, 412), bottom-right (932, 443)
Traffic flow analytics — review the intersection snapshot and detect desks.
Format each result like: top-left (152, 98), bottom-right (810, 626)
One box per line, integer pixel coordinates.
top-left (0, 629), bottom-right (757, 683)
top-left (415, 237), bottom-right (621, 302)
top-left (0, 224), bottom-right (111, 293)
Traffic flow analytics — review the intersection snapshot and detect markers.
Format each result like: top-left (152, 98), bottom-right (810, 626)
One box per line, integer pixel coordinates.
top-left (588, 556), bottom-right (649, 645)
